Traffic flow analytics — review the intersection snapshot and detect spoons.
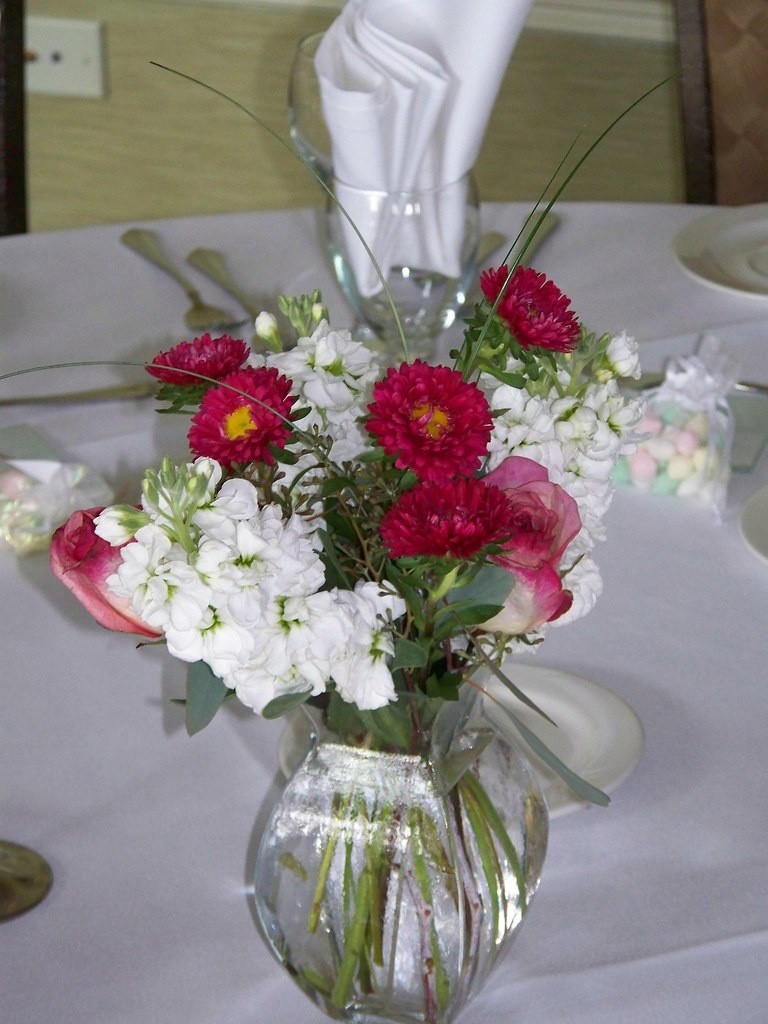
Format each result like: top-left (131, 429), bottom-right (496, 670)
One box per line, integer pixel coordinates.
top-left (121, 229), bottom-right (246, 331)
top-left (186, 248), bottom-right (298, 354)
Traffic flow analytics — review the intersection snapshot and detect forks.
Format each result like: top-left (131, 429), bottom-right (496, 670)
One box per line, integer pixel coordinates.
top-left (582, 359), bottom-right (768, 395)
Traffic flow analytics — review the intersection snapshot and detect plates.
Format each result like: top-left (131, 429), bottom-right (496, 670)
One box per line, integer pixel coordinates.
top-left (738, 481), bottom-right (768, 567)
top-left (671, 203), bottom-right (768, 300)
top-left (279, 664), bottom-right (645, 818)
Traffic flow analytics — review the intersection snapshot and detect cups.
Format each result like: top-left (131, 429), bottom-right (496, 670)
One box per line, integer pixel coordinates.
top-left (290, 30), bottom-right (334, 193)
top-left (326, 169), bottom-right (480, 369)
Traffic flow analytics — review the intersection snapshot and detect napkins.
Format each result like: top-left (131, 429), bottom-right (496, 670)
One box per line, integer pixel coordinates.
top-left (313, 0), bottom-right (533, 296)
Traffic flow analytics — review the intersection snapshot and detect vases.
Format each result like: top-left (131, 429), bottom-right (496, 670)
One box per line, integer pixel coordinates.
top-left (254, 634), bottom-right (550, 1024)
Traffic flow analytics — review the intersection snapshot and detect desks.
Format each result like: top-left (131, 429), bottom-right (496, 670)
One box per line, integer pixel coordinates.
top-left (0, 205), bottom-right (768, 1024)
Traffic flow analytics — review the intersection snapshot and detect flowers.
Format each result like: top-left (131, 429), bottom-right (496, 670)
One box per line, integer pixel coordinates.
top-left (0, 55), bottom-right (693, 1018)
